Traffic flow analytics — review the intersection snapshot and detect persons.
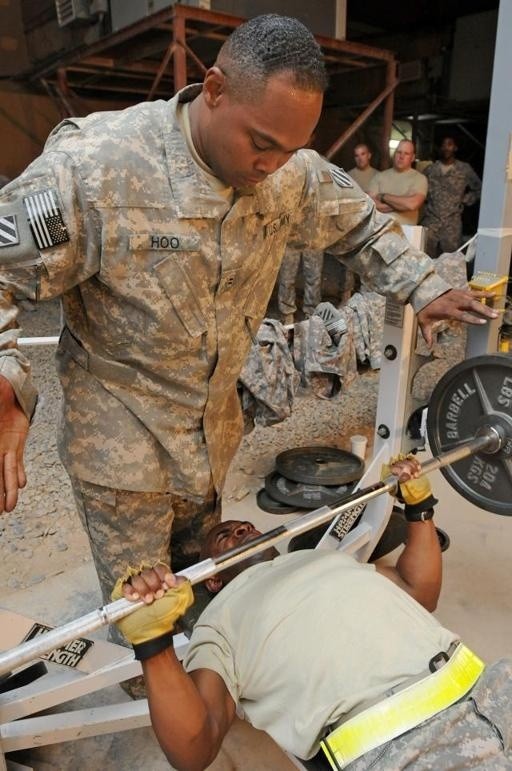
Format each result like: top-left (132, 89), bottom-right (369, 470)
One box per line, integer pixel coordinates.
top-left (368, 138), bottom-right (430, 243)
top-left (1, 9), bottom-right (499, 706)
top-left (341, 142), bottom-right (382, 304)
top-left (276, 132), bottom-right (327, 327)
top-left (418, 134), bottom-right (483, 260)
top-left (106, 455), bottom-right (512, 771)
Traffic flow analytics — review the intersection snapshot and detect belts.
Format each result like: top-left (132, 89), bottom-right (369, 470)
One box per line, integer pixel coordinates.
top-left (318, 641), bottom-right (487, 771)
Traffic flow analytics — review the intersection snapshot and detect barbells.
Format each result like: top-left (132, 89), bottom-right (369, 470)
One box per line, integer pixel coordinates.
top-left (0, 355), bottom-right (511, 678)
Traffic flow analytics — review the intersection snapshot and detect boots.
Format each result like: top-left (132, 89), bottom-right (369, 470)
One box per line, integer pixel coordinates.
top-left (120, 675), bottom-right (147, 699)
top-left (281, 313), bottom-right (294, 339)
